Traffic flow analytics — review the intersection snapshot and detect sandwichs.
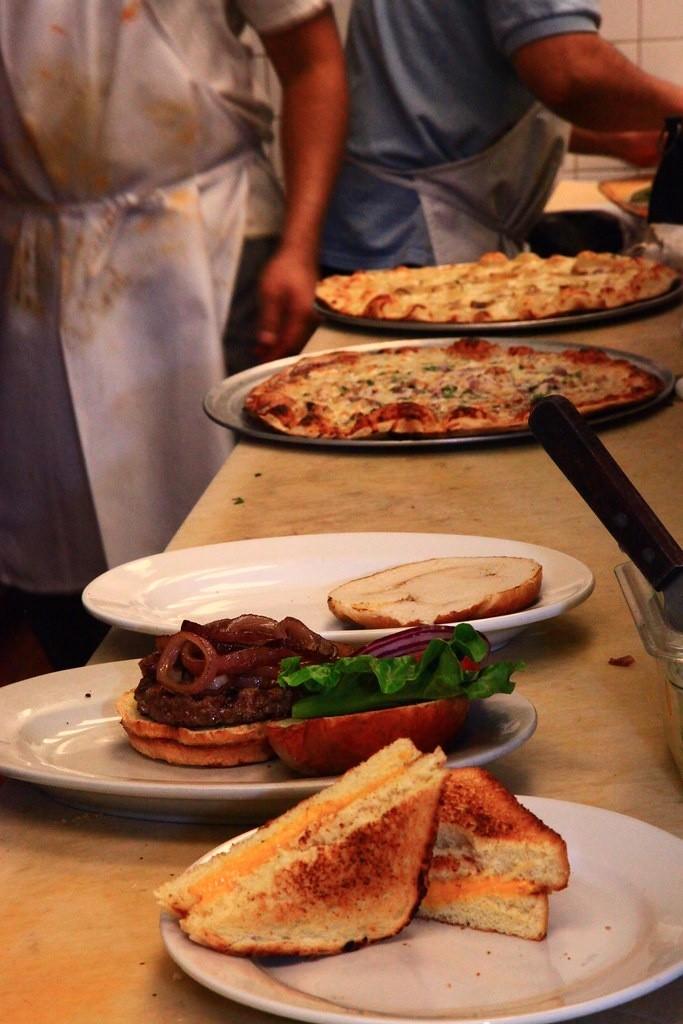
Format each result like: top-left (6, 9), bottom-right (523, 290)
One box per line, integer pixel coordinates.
top-left (166, 735), bottom-right (569, 961)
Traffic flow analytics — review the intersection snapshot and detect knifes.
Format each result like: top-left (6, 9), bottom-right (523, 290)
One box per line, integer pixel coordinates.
top-left (525, 392), bottom-right (680, 638)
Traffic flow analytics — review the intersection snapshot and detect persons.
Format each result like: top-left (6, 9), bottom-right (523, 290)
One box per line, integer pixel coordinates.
top-left (0, 0), bottom-right (683, 688)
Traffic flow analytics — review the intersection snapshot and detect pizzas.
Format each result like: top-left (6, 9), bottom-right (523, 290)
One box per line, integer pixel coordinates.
top-left (245, 252), bottom-right (677, 441)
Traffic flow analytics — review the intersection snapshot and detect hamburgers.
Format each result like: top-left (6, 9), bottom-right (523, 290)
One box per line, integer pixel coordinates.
top-left (115, 554), bottom-right (544, 774)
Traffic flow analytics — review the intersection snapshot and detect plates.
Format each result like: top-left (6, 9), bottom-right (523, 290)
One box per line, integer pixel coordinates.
top-left (79, 532), bottom-right (594, 654)
top-left (0, 654), bottom-right (537, 817)
top-left (599, 173), bottom-right (657, 218)
top-left (202, 338), bottom-right (673, 447)
top-left (157, 790), bottom-right (683, 1024)
top-left (310, 248), bottom-right (683, 332)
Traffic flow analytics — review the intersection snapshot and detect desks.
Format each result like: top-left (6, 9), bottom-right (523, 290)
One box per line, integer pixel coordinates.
top-left (0, 177), bottom-right (682, 1024)
